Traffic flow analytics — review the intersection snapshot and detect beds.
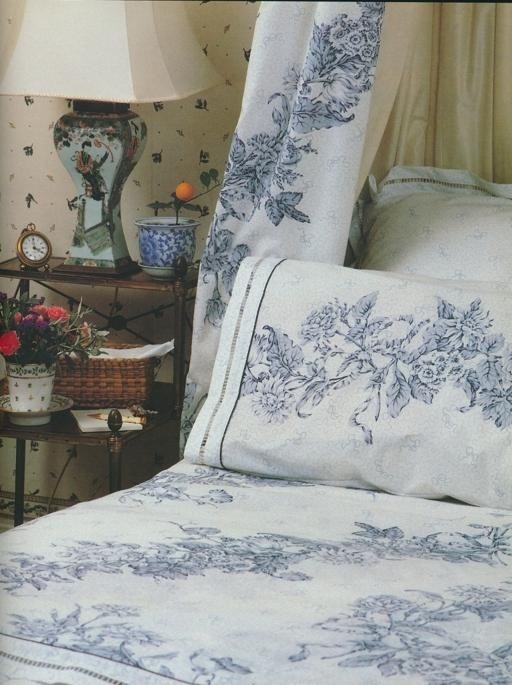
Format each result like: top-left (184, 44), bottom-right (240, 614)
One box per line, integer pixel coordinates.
top-left (0, 0), bottom-right (512, 685)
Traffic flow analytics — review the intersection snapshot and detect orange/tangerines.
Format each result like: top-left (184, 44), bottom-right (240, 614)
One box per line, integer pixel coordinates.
top-left (175, 182), bottom-right (193, 201)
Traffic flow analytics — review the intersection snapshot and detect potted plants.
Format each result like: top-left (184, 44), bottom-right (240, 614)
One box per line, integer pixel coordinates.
top-left (131, 166), bottom-right (226, 278)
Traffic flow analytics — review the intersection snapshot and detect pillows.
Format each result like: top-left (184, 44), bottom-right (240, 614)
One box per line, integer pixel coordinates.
top-left (183, 256), bottom-right (512, 512)
top-left (342, 164), bottom-right (512, 291)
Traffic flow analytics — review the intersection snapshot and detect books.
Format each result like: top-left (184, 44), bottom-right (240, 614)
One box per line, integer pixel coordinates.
top-left (71, 407), bottom-right (143, 435)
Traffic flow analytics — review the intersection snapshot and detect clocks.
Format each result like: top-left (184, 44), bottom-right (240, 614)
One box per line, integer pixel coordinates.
top-left (17, 221), bottom-right (54, 271)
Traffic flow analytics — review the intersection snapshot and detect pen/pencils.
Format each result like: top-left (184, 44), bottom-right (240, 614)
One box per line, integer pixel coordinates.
top-left (87, 414), bottom-right (146, 424)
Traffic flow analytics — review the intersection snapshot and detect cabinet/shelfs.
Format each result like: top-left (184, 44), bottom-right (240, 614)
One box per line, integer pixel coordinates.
top-left (0, 253), bottom-right (202, 531)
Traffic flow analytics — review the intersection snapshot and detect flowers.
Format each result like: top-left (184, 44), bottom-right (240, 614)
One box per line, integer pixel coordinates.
top-left (0, 287), bottom-right (112, 377)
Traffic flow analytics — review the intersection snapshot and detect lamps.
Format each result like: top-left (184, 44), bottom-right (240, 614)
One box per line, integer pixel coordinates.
top-left (0, 0), bottom-right (229, 278)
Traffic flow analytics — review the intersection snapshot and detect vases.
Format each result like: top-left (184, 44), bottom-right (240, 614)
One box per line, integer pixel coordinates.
top-left (0, 361), bottom-right (74, 428)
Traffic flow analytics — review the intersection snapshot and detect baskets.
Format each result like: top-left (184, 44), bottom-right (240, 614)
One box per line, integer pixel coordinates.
top-left (4, 342), bottom-right (155, 410)
